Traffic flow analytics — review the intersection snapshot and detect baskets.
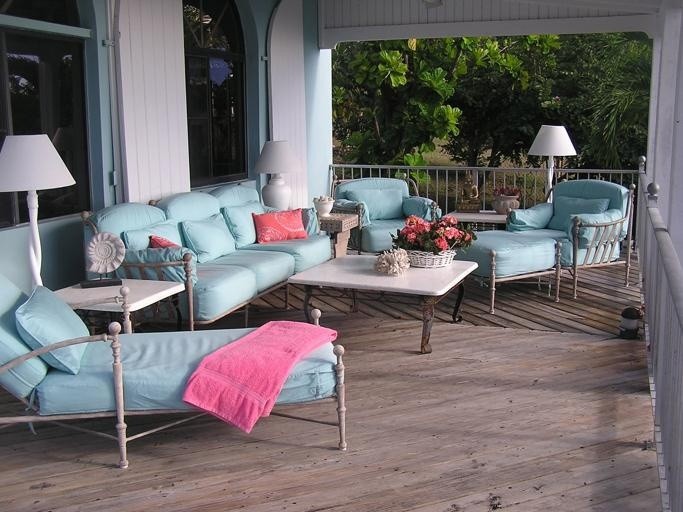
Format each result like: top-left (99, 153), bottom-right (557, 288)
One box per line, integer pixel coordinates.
top-left (406, 249), bottom-right (457, 268)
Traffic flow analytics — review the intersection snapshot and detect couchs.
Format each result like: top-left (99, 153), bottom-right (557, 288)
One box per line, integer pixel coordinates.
top-left (451, 230), bottom-right (561, 314)
top-left (78, 184), bottom-right (330, 330)
top-left (334, 177), bottom-right (438, 253)
top-left (504, 178), bottom-right (636, 300)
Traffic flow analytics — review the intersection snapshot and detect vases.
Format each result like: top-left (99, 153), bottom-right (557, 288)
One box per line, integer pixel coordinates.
top-left (490, 196), bottom-right (519, 215)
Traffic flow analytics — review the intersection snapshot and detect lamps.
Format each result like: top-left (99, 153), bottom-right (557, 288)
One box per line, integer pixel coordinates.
top-left (254, 140), bottom-right (301, 210)
top-left (528, 124), bottom-right (576, 201)
top-left (618, 306), bottom-right (640, 338)
top-left (0, 134), bottom-right (77, 289)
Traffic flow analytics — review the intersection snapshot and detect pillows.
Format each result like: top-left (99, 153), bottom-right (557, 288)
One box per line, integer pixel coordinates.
top-left (545, 196), bottom-right (609, 231)
top-left (123, 219), bottom-right (181, 248)
top-left (220, 200), bottom-right (264, 248)
top-left (149, 234), bottom-right (180, 248)
top-left (14, 285), bottom-right (91, 376)
top-left (180, 213), bottom-right (236, 263)
top-left (345, 186), bottom-right (406, 220)
top-left (251, 208), bottom-right (306, 243)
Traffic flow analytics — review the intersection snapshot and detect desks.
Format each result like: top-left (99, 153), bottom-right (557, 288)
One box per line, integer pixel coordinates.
top-left (441, 209), bottom-right (507, 231)
top-left (315, 212), bottom-right (358, 257)
top-left (51, 278), bottom-right (186, 335)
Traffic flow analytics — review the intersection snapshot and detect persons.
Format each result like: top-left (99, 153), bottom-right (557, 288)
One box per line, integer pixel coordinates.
top-left (457, 174), bottom-right (481, 213)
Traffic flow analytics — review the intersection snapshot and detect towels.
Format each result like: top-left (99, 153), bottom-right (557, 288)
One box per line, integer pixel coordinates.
top-left (181, 319), bottom-right (338, 435)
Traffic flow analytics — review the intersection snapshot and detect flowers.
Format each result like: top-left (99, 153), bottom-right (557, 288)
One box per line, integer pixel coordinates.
top-left (491, 186), bottom-right (521, 196)
top-left (389, 213), bottom-right (477, 253)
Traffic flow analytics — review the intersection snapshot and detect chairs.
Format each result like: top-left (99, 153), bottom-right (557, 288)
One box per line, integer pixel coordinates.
top-left (0, 275), bottom-right (348, 470)
